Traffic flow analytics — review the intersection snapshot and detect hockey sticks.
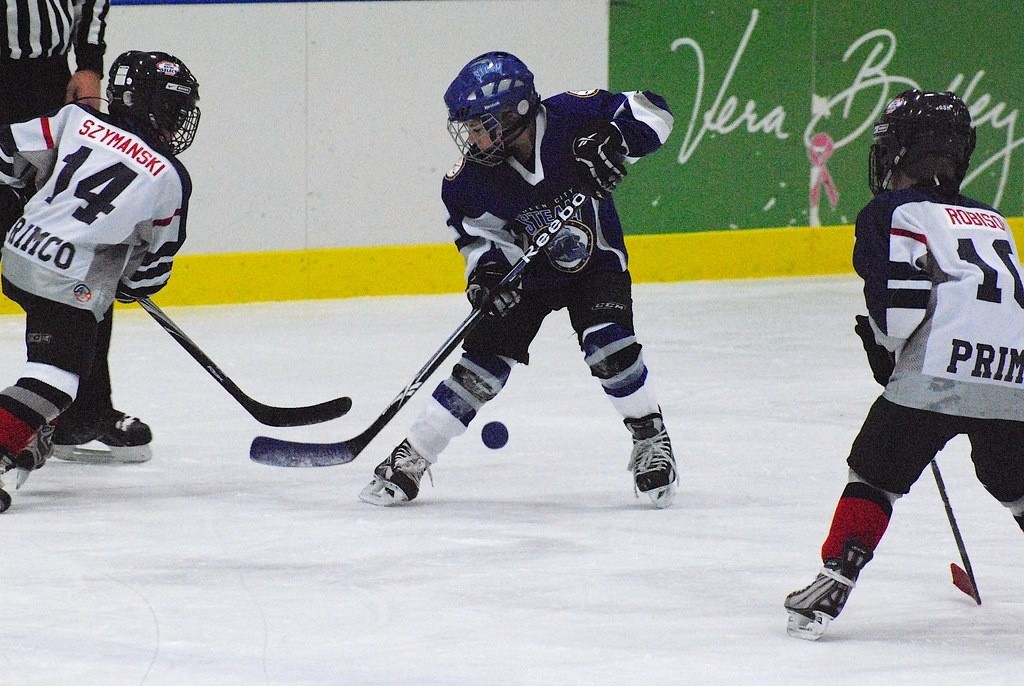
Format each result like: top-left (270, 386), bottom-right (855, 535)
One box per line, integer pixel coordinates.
top-left (931, 457), bottom-right (982, 606)
top-left (135, 296), bottom-right (352, 427)
top-left (249, 151), bottom-right (626, 468)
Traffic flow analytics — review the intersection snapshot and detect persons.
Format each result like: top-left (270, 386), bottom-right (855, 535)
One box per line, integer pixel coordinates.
top-left (0, 49), bottom-right (199, 512)
top-left (359, 50), bottom-right (679, 509)
top-left (783, 89), bottom-right (1024, 641)
top-left (0, 1), bottom-right (153, 461)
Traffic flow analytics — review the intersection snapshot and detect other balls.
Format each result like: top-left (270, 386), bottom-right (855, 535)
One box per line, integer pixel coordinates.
top-left (481, 421), bottom-right (509, 449)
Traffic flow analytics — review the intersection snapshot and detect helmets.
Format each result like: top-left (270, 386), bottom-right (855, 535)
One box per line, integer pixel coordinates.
top-left (444, 52), bottom-right (541, 168)
top-left (867, 89), bottom-right (978, 193)
top-left (106, 50), bottom-right (201, 158)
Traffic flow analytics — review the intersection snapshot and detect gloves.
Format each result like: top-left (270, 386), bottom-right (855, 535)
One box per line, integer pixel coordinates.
top-left (853, 316), bottom-right (895, 387)
top-left (569, 123), bottom-right (630, 203)
top-left (466, 251), bottom-right (524, 317)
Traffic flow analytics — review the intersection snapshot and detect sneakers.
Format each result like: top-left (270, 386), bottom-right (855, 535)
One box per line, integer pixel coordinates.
top-left (622, 404), bottom-right (676, 509)
top-left (360, 439), bottom-right (435, 508)
top-left (0, 446), bottom-right (17, 511)
top-left (50, 402), bottom-right (154, 467)
top-left (781, 541), bottom-right (873, 641)
